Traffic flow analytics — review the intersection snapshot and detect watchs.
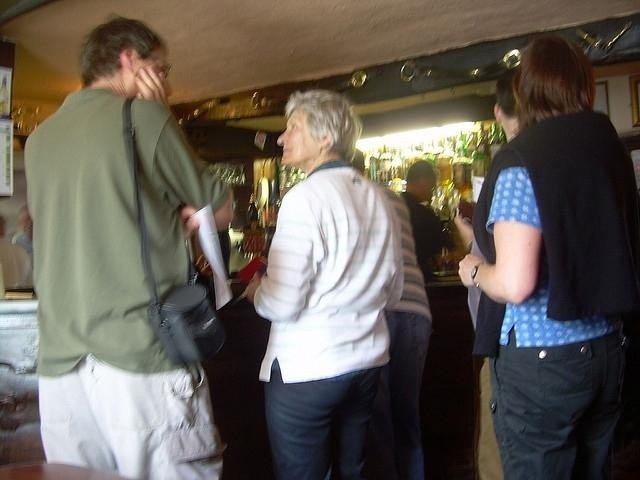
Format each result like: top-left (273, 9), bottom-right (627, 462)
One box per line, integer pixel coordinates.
top-left (472, 262), bottom-right (484, 286)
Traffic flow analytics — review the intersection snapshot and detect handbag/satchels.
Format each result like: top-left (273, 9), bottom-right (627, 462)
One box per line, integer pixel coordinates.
top-left (145, 282), bottom-right (227, 367)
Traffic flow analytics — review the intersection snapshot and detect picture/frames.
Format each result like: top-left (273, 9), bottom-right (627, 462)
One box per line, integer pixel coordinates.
top-left (591, 74), bottom-right (640, 127)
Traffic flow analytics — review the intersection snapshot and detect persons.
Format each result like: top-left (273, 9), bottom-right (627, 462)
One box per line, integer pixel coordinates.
top-left (453, 70), bottom-right (520, 478)
top-left (345, 148), bottom-right (436, 478)
top-left (1, 214), bottom-right (31, 294)
top-left (12, 203), bottom-right (33, 255)
top-left (245, 89), bottom-right (406, 477)
top-left (399, 159), bottom-right (452, 271)
top-left (459, 32), bottom-right (638, 479)
top-left (23, 15), bottom-right (235, 478)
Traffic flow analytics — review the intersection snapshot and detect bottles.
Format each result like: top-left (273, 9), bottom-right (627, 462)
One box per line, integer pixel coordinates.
top-left (459, 164), bottom-right (475, 224)
top-left (248, 200), bottom-right (260, 231)
top-left (472, 122), bottom-right (508, 176)
